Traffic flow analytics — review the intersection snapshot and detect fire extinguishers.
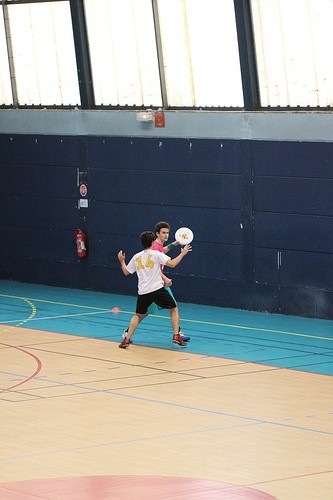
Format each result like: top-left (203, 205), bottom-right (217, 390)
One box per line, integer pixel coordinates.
top-left (76, 229), bottom-right (86, 257)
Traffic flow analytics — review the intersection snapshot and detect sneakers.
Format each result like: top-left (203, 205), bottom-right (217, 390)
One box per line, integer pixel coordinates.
top-left (181, 335), bottom-right (190, 341)
top-left (172, 333), bottom-right (187, 345)
top-left (122, 329), bottom-right (129, 338)
top-left (118, 339), bottom-right (132, 348)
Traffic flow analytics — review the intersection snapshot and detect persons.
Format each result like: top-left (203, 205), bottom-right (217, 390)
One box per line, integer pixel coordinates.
top-left (122, 221), bottom-right (190, 341)
top-left (118, 231), bottom-right (192, 348)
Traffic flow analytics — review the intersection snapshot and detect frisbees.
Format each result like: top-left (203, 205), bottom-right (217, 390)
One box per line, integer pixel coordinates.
top-left (175, 227), bottom-right (194, 245)
